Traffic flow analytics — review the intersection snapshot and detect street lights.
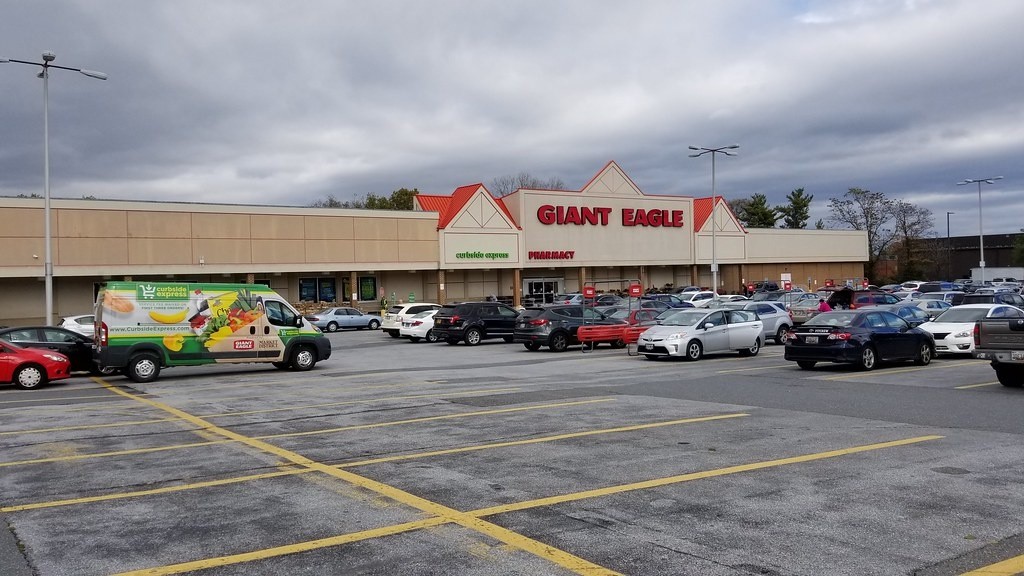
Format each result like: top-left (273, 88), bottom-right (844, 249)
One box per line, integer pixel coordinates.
top-left (688, 143), bottom-right (739, 308)
top-left (957, 175), bottom-right (1004, 284)
top-left (947, 212), bottom-right (954, 282)
top-left (0, 51), bottom-right (107, 340)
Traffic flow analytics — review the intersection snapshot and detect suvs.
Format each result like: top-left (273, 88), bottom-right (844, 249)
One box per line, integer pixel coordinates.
top-left (381, 303), bottom-right (443, 338)
top-left (513, 304), bottom-right (629, 352)
top-left (430, 301), bottom-right (520, 346)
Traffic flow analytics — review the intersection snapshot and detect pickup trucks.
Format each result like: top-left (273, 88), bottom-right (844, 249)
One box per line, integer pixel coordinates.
top-left (971, 316), bottom-right (1024, 387)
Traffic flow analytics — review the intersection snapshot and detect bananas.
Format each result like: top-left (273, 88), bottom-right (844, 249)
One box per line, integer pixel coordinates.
top-left (149, 307), bottom-right (190, 324)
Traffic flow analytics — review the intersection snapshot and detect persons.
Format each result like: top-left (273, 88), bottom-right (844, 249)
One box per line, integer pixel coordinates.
top-left (818, 299), bottom-right (832, 312)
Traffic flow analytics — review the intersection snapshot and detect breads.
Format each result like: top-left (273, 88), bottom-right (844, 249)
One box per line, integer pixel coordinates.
top-left (103, 290), bottom-right (134, 313)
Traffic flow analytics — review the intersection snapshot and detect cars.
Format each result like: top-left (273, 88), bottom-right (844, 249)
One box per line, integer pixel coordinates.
top-left (400, 310), bottom-right (440, 343)
top-left (783, 309), bottom-right (935, 372)
top-left (553, 277), bottom-right (1024, 359)
top-left (305, 307), bottom-right (382, 332)
top-left (637, 309), bottom-right (765, 362)
top-left (0, 314), bottom-right (116, 391)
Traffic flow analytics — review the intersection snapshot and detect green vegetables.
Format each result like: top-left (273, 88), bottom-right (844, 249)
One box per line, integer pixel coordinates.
top-left (197, 314), bottom-right (230, 341)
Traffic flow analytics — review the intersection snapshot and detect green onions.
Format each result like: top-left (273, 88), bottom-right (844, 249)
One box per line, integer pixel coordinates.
top-left (233, 288), bottom-right (258, 313)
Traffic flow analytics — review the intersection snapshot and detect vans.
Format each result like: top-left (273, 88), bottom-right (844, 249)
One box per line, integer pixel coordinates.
top-left (91, 281), bottom-right (332, 383)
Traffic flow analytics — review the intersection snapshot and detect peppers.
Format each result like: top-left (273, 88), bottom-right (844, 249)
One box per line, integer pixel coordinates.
top-left (228, 306), bottom-right (262, 333)
top-left (163, 333), bottom-right (187, 352)
